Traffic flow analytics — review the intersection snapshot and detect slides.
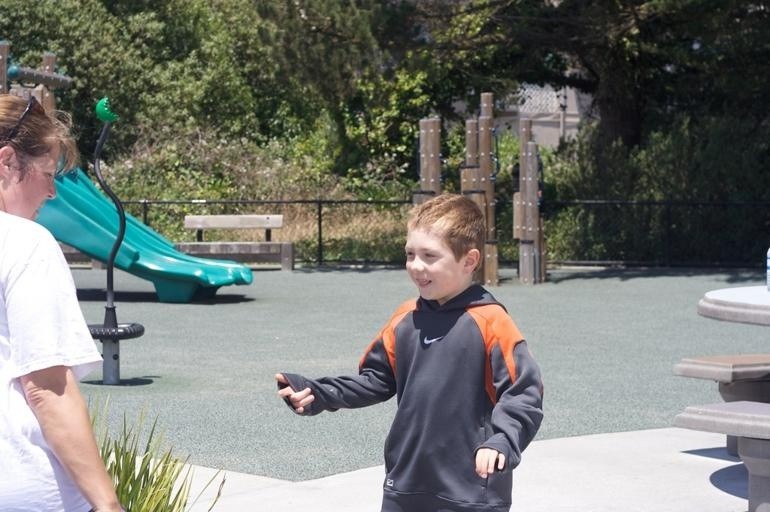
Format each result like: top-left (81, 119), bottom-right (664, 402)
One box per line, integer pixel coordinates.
top-left (33, 157), bottom-right (253, 305)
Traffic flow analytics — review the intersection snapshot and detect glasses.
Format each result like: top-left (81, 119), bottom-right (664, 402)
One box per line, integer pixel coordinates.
top-left (6, 94), bottom-right (46, 148)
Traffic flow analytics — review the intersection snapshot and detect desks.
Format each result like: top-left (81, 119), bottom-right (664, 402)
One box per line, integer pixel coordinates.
top-left (698, 285), bottom-right (770, 326)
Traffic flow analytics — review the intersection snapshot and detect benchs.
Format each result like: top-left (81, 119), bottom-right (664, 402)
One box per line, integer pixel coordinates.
top-left (173, 214), bottom-right (294, 271)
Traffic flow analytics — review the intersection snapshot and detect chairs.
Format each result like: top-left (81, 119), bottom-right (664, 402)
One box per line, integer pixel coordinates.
top-left (673, 401), bottom-right (770, 512)
top-left (673, 353), bottom-right (770, 457)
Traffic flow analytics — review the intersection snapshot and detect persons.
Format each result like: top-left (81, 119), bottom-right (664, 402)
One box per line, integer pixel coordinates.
top-left (274, 191), bottom-right (546, 512)
top-left (0, 92), bottom-right (136, 512)
